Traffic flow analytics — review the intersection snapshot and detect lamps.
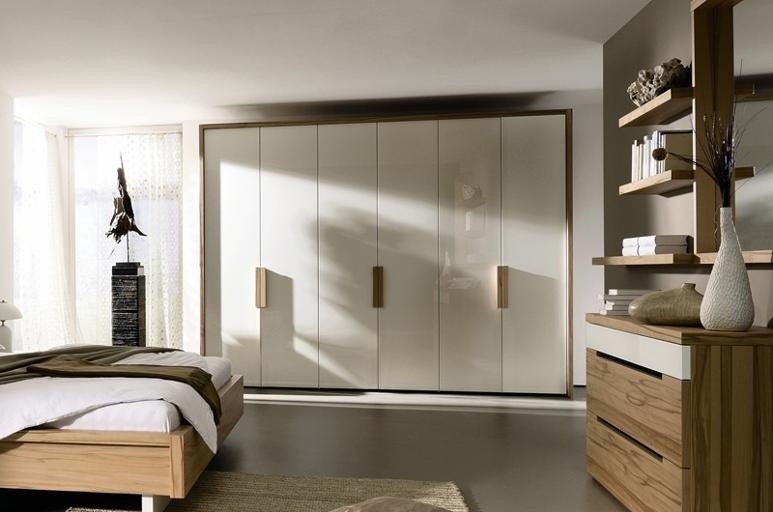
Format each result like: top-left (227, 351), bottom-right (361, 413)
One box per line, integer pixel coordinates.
top-left (0, 299), bottom-right (23, 353)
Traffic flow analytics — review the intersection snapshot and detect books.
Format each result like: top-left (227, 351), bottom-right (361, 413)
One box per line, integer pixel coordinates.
top-left (621, 234), bottom-right (689, 257)
top-left (598, 289), bottom-right (660, 316)
top-left (632, 129), bottom-right (693, 183)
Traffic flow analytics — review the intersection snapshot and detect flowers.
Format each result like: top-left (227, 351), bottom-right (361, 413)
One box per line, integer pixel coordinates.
top-left (652, 61), bottom-right (772, 207)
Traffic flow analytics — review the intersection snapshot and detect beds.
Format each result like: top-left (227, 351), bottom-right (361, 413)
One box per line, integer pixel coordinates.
top-left (0, 345), bottom-right (247, 512)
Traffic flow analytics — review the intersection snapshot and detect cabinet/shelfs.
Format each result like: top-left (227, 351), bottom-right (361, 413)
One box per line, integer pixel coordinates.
top-left (439, 114), bottom-right (568, 395)
top-left (203, 123), bottom-right (318, 389)
top-left (319, 118), bottom-right (439, 391)
top-left (591, 81), bottom-right (773, 271)
top-left (584, 314), bottom-right (773, 511)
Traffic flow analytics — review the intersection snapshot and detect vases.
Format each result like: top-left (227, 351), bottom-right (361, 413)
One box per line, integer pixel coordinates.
top-left (700, 207), bottom-right (756, 331)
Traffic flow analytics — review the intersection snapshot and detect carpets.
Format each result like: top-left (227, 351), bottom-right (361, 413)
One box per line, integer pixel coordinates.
top-left (64, 471), bottom-right (470, 512)
